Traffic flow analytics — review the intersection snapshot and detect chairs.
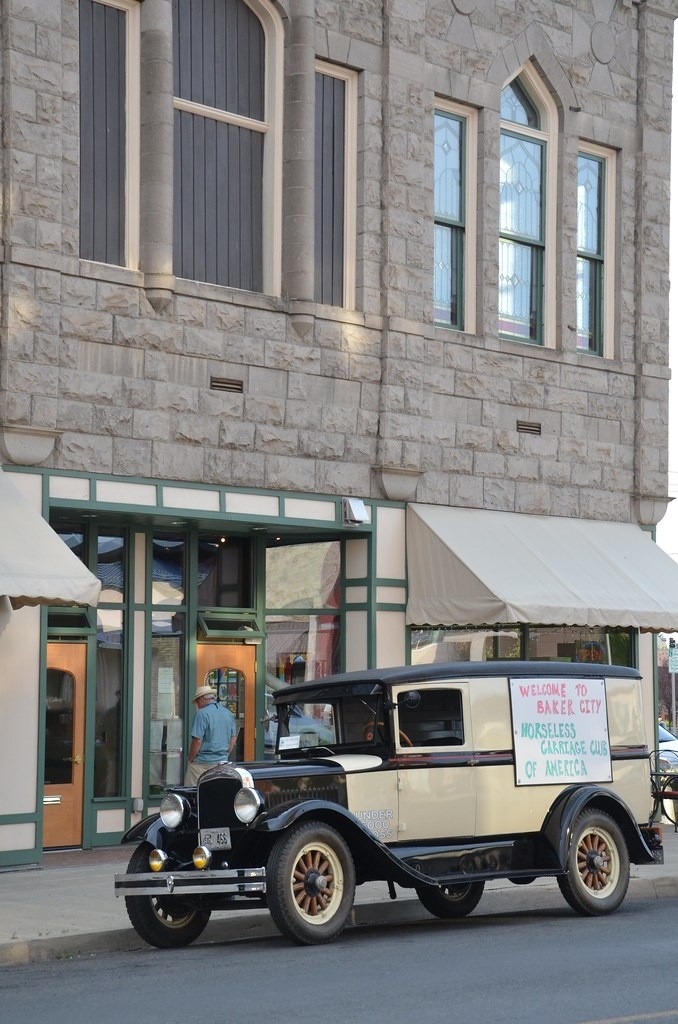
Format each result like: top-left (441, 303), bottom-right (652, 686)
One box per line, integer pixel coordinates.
top-left (648, 749), bottom-right (678, 832)
top-left (422, 736), bottom-right (464, 747)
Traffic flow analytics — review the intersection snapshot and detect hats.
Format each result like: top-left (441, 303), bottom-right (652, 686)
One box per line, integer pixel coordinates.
top-left (192, 686), bottom-right (217, 700)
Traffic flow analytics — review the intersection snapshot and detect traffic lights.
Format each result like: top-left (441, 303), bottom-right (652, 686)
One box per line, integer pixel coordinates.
top-left (669, 638), bottom-right (675, 648)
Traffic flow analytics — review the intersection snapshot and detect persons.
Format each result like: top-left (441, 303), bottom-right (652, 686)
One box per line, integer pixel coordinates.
top-left (184, 685), bottom-right (236, 790)
top-left (98, 689), bottom-right (120, 796)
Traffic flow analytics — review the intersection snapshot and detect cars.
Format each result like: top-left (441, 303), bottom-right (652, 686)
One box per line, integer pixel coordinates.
top-left (264, 692), bottom-right (334, 749)
top-left (658, 725), bottom-right (678, 771)
top-left (114, 662), bottom-right (665, 949)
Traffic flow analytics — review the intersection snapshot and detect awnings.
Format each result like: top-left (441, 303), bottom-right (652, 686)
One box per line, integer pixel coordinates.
top-left (0, 467), bottom-right (102, 610)
top-left (404, 499), bottom-right (678, 637)
